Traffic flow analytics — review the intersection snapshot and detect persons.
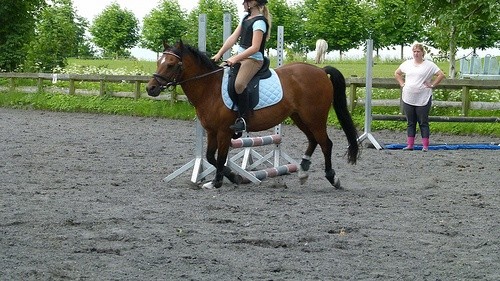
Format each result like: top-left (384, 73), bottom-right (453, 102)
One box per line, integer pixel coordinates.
top-left (211, 0), bottom-right (271, 129)
top-left (394, 44), bottom-right (444, 152)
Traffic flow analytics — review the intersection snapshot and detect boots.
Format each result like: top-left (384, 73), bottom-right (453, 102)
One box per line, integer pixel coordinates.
top-left (229, 86), bottom-right (249, 130)
top-left (422, 138), bottom-right (429, 151)
top-left (403, 137), bottom-right (414, 150)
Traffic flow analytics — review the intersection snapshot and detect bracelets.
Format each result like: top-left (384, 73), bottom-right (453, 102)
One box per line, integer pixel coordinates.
top-left (431, 83), bottom-right (434, 87)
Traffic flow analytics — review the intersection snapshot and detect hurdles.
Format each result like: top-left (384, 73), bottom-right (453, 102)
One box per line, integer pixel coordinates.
top-left (348, 38), bottom-right (500, 149)
top-left (195, 12), bottom-right (301, 183)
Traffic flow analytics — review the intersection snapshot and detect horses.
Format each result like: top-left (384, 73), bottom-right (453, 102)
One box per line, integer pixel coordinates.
top-left (146, 37), bottom-right (361, 190)
